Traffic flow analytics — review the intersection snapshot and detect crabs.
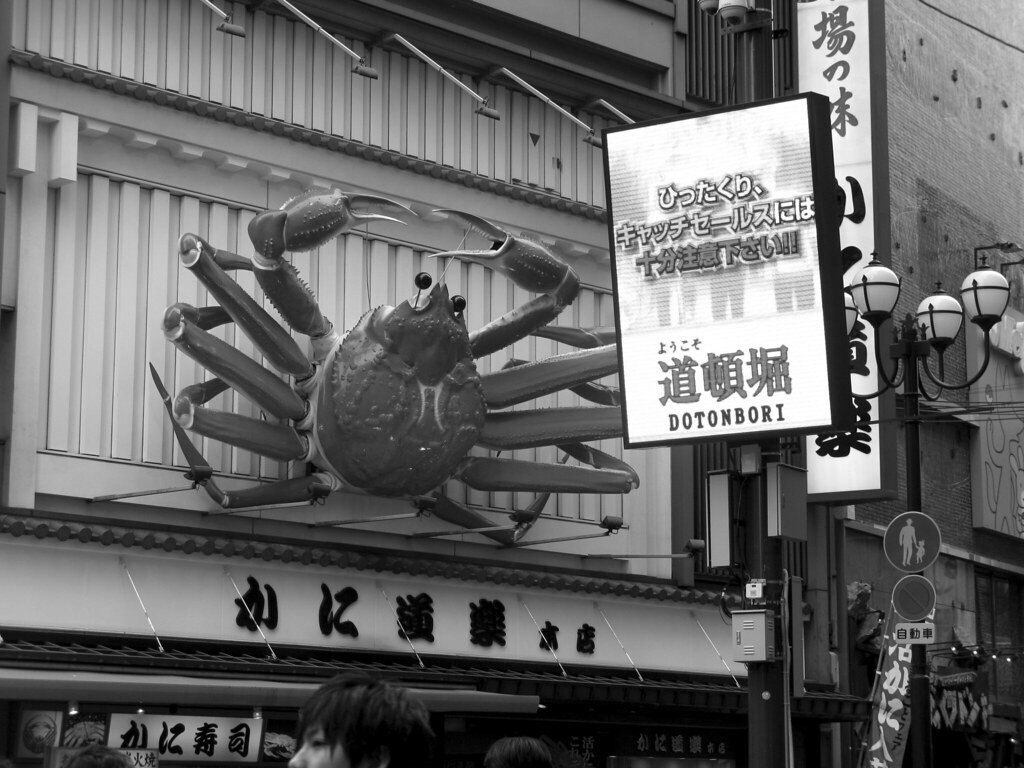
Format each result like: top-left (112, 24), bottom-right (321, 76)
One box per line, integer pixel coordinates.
top-left (163, 190), bottom-right (640, 544)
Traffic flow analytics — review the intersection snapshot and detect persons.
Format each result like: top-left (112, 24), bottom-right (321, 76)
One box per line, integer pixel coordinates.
top-left (288, 674), bottom-right (437, 768)
top-left (62, 744), bottom-right (134, 768)
top-left (484, 736), bottom-right (551, 768)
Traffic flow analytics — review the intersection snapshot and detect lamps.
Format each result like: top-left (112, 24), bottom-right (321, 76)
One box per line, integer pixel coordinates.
top-left (68, 701), bottom-right (79, 715)
top-left (279, 0), bottom-right (378, 80)
top-left (315, 495), bottom-right (438, 527)
top-left (209, 482), bottom-right (332, 515)
top-left (253, 708), bottom-right (263, 720)
top-left (384, 35), bottom-right (502, 122)
top-left (90, 465), bottom-right (213, 504)
top-left (411, 510), bottom-right (535, 538)
top-left (486, 68), bottom-right (603, 149)
top-left (497, 515), bottom-right (624, 551)
top-left (200, 0), bottom-right (247, 38)
top-left (586, 538), bottom-right (705, 561)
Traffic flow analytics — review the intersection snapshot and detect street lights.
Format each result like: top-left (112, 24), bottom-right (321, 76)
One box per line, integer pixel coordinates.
top-left (841, 251), bottom-right (1010, 768)
